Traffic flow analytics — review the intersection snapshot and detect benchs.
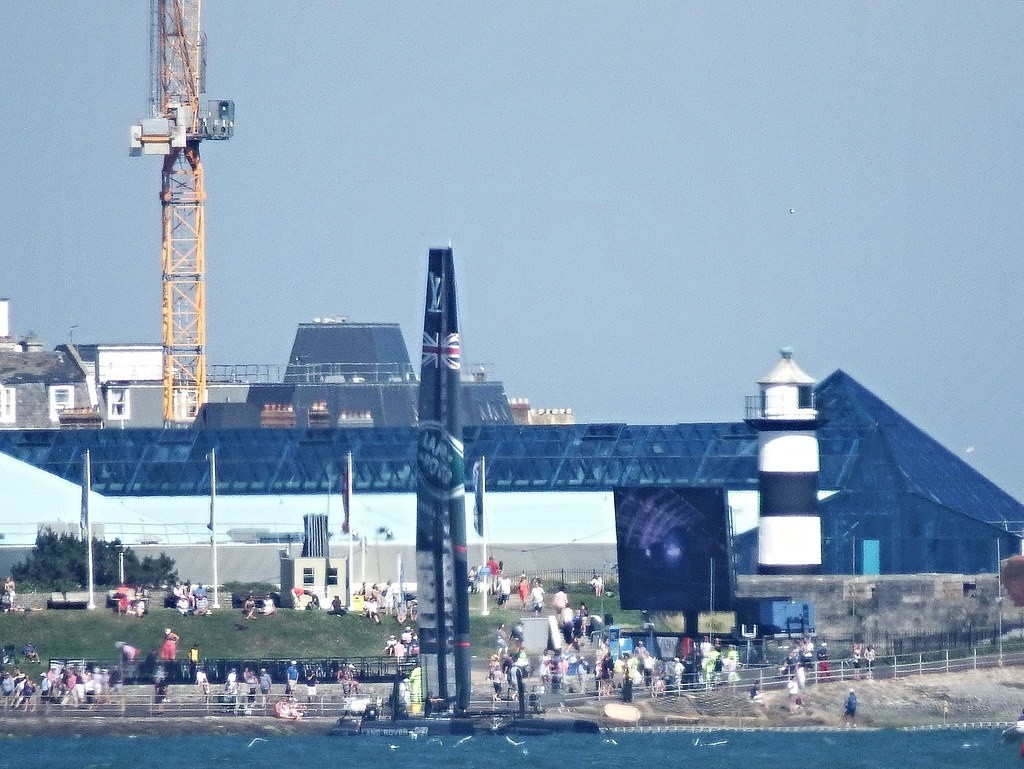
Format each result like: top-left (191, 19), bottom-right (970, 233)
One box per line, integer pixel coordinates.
top-left (50, 590), bottom-right (89, 609)
top-left (109, 589), bottom-right (152, 611)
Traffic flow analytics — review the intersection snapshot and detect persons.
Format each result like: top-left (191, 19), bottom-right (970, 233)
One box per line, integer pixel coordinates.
top-left (852, 644), bottom-right (863, 680)
top-left (327, 595), bottom-right (348, 619)
top-left (0, 627), bottom-right (424, 719)
top-left (750, 681), bottom-right (764, 706)
top-left (551, 586), bottom-right (568, 611)
top-left (786, 676), bottom-right (800, 711)
top-left (515, 574), bottom-right (530, 611)
top-left (169, 580), bottom-right (209, 615)
top-left (467, 555), bottom-right (510, 609)
top-left (778, 631), bottom-right (831, 690)
top-left (0, 574), bottom-right (20, 612)
top-left (356, 579), bottom-right (418, 624)
top-left (188, 644), bottom-right (200, 664)
top-left (1001, 708), bottom-right (1024, 758)
top-left (843, 687), bottom-right (856, 723)
top-left (864, 645), bottom-right (875, 679)
top-left (530, 581), bottom-right (545, 617)
top-left (590, 574), bottom-right (602, 597)
top-left (290, 586), bottom-right (320, 611)
top-left (108, 577), bottom-right (150, 617)
top-left (160, 628), bottom-right (179, 660)
top-left (487, 602), bottom-right (742, 698)
top-left (245, 594), bottom-right (276, 620)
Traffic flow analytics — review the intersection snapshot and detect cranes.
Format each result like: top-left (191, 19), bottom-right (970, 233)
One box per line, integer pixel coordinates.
top-left (124, 0), bottom-right (238, 426)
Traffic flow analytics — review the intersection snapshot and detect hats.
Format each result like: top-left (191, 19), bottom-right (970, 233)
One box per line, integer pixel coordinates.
top-left (491, 654), bottom-right (498, 660)
top-left (674, 658), bottom-right (679, 662)
top-left (40, 672), bottom-right (47, 678)
top-left (821, 643), bottom-right (828, 646)
top-left (520, 574), bottom-right (526, 578)
top-left (559, 586), bottom-right (565, 590)
top-left (390, 635), bottom-right (395, 639)
top-left (165, 629), bottom-right (171, 634)
top-left (569, 658), bottom-right (577, 664)
top-left (349, 664), bottom-right (355, 668)
top-left (291, 661), bottom-right (297, 665)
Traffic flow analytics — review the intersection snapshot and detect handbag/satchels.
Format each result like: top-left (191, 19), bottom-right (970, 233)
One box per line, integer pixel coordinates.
top-left (112, 593), bottom-right (124, 599)
top-left (135, 648), bottom-right (141, 655)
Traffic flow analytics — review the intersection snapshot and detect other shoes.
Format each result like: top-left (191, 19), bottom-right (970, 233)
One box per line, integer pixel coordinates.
top-left (511, 696), bottom-right (519, 701)
top-left (521, 608), bottom-right (526, 611)
top-left (622, 698), bottom-right (632, 703)
top-left (288, 697), bottom-right (298, 702)
top-left (855, 676), bottom-right (860, 680)
top-left (494, 695), bottom-right (501, 701)
